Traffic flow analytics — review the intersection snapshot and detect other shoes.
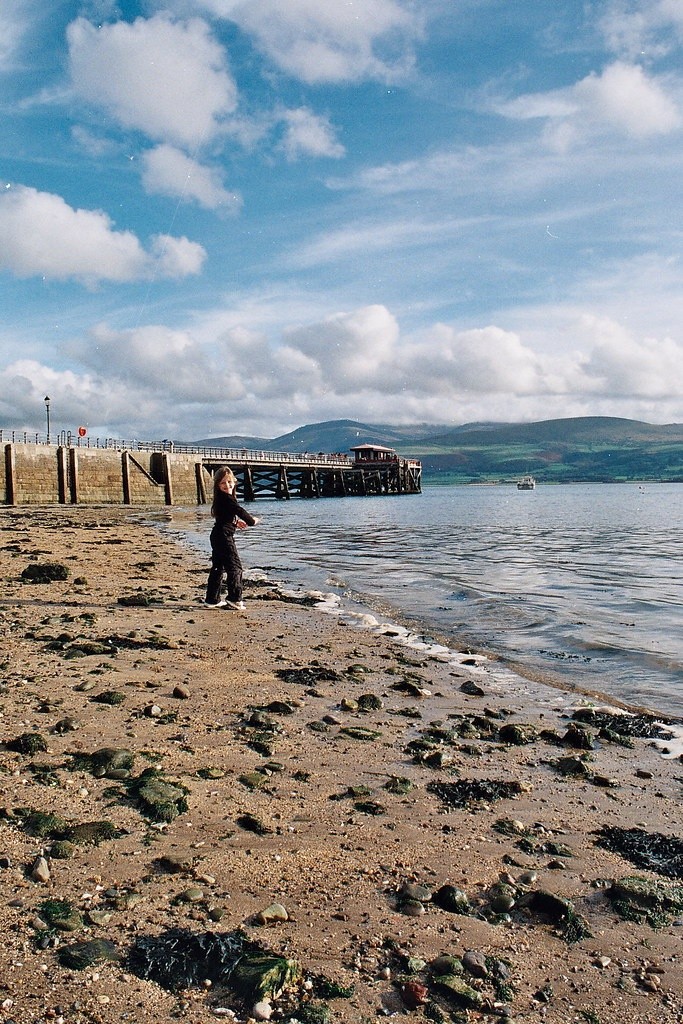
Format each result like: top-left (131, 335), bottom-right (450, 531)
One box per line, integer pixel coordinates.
top-left (225, 598), bottom-right (247, 610)
top-left (204, 601), bottom-right (227, 607)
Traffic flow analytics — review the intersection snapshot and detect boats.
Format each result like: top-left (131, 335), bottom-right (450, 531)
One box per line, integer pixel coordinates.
top-left (517, 476), bottom-right (535, 491)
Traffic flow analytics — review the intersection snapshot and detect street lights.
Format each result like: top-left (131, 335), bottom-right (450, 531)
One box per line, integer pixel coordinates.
top-left (44, 396), bottom-right (51, 445)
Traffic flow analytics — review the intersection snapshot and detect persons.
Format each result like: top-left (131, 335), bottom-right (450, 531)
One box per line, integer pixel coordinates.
top-left (303, 450), bottom-right (350, 462)
top-left (204, 467), bottom-right (258, 609)
